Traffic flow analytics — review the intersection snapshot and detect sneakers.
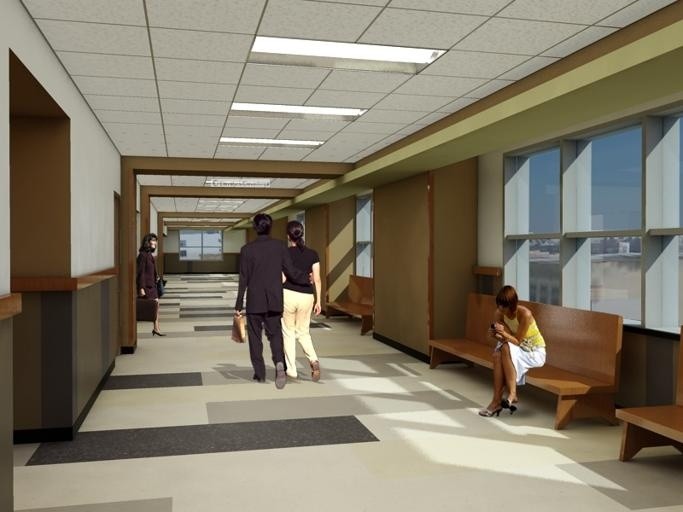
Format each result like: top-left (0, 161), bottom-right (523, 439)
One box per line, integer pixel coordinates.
top-left (253, 372), bottom-right (266, 383)
top-left (310, 360), bottom-right (320, 382)
top-left (275, 361), bottom-right (286, 389)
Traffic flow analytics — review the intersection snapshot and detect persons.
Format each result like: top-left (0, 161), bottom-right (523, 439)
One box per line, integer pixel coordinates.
top-left (134, 232), bottom-right (167, 337)
top-left (231, 212), bottom-right (314, 390)
top-left (477, 283), bottom-right (547, 417)
top-left (278, 219), bottom-right (321, 383)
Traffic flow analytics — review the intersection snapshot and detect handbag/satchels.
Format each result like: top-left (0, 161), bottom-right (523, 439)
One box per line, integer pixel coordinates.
top-left (230, 313), bottom-right (248, 344)
top-left (136, 294), bottom-right (158, 323)
top-left (156, 278), bottom-right (167, 299)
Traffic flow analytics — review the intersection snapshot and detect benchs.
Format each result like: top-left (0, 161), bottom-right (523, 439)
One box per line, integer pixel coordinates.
top-left (612, 324), bottom-right (682, 462)
top-left (324, 275), bottom-right (375, 338)
top-left (428, 291), bottom-right (626, 432)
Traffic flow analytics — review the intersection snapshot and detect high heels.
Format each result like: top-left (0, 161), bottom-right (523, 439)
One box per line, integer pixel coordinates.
top-left (152, 330), bottom-right (167, 337)
top-left (500, 399), bottom-right (518, 416)
top-left (479, 404), bottom-right (504, 417)
